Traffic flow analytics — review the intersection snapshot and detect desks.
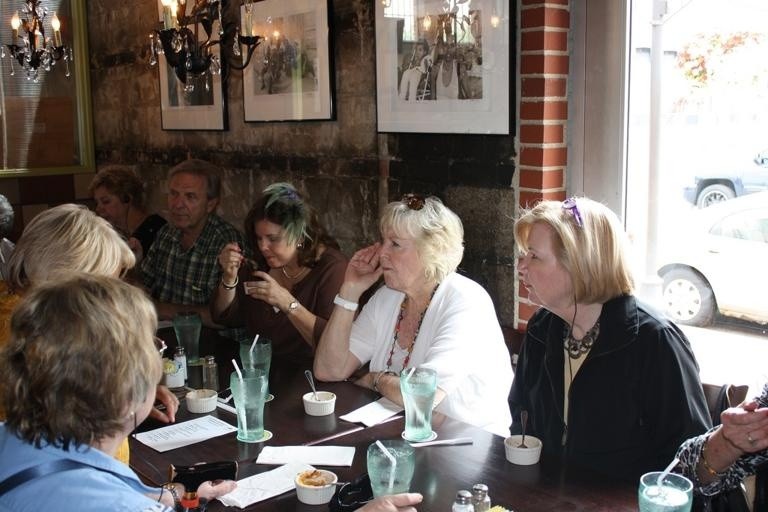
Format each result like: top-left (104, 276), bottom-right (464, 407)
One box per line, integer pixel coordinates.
top-left (123, 313), bottom-right (690, 511)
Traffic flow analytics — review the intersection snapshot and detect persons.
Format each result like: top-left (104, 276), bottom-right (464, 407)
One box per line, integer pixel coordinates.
top-left (665, 347), bottom-right (767, 511)
top-left (401, 32), bottom-right (483, 101)
top-left (0, 191), bottom-right (420, 510)
top-left (84, 164), bottom-right (516, 440)
top-left (504, 194), bottom-right (713, 486)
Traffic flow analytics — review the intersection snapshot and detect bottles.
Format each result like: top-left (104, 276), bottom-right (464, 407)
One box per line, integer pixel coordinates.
top-left (452, 482), bottom-right (492, 512)
top-left (202, 356), bottom-right (219, 389)
top-left (172, 347), bottom-right (189, 381)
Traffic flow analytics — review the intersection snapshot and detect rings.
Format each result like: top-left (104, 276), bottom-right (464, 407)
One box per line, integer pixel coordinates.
top-left (747, 432), bottom-right (756, 448)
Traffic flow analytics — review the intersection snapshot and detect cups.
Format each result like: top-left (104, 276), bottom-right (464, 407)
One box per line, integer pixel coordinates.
top-left (401, 367), bottom-right (437, 440)
top-left (241, 338), bottom-right (272, 400)
top-left (366, 439), bottom-right (415, 500)
top-left (295, 470), bottom-right (337, 504)
top-left (302, 391), bottom-right (337, 416)
top-left (186, 389), bottom-right (218, 413)
top-left (505, 435), bottom-right (543, 465)
top-left (638, 471), bottom-right (694, 511)
top-left (174, 310), bottom-right (202, 364)
top-left (230, 369), bottom-right (269, 440)
top-left (243, 281), bottom-right (260, 295)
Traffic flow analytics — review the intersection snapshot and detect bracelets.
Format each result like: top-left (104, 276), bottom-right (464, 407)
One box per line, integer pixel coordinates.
top-left (699, 444), bottom-right (727, 477)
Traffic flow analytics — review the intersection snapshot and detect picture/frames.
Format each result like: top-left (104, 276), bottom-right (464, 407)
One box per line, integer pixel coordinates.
top-left (236, 0), bottom-right (338, 125)
top-left (375, 0), bottom-right (516, 139)
top-left (154, 0), bottom-right (229, 133)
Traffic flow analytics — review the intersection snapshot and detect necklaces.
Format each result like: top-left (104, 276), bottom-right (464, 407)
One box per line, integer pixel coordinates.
top-left (561, 318), bottom-right (600, 360)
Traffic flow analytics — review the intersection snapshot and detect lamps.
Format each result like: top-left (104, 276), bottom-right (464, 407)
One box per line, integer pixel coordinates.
top-left (1, 2), bottom-right (74, 88)
top-left (139, 0), bottom-right (265, 92)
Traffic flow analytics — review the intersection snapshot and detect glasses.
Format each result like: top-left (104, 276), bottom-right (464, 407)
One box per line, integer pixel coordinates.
top-left (401, 192), bottom-right (426, 211)
top-left (328, 474), bottom-right (375, 511)
top-left (563, 195), bottom-right (584, 230)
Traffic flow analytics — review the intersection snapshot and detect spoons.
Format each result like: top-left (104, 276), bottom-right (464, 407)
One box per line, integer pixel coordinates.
top-left (517, 410), bottom-right (530, 448)
top-left (304, 478), bottom-right (345, 486)
top-left (305, 370), bottom-right (322, 400)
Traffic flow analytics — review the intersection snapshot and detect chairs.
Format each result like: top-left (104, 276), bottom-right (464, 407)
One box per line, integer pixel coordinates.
top-left (687, 382), bottom-right (745, 512)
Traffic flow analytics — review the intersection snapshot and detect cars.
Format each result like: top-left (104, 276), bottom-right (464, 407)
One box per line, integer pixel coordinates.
top-left (650, 190), bottom-right (768, 335)
top-left (682, 142), bottom-right (767, 208)
